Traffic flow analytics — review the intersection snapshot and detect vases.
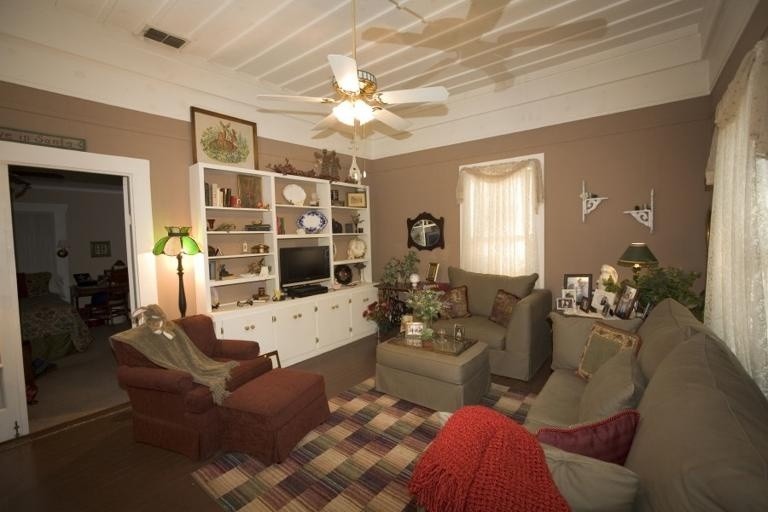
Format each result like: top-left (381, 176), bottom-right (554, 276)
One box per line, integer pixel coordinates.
top-left (422, 338), bottom-right (433, 348)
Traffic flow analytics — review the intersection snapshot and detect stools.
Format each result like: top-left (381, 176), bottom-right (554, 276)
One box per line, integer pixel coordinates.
top-left (221, 367), bottom-right (335, 461)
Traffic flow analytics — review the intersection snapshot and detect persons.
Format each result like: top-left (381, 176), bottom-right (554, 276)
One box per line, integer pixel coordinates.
top-left (620, 292), bottom-right (631, 314)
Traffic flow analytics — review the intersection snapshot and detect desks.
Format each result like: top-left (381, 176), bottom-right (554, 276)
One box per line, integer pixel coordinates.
top-left (68, 277), bottom-right (129, 325)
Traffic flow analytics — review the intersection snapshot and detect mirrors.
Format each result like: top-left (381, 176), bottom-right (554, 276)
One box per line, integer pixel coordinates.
top-left (406, 212), bottom-right (445, 251)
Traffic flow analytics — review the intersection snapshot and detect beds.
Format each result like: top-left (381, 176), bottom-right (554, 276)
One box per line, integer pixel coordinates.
top-left (18, 272), bottom-right (92, 358)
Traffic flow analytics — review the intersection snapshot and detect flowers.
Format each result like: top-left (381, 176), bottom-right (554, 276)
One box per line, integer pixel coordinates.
top-left (362, 301), bottom-right (393, 332)
top-left (382, 257), bottom-right (400, 287)
top-left (408, 290), bottom-right (443, 320)
top-left (421, 328), bottom-right (434, 341)
top-left (401, 252), bottom-right (420, 282)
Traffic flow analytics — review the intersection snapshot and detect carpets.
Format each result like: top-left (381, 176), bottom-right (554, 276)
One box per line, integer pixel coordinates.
top-left (190, 367), bottom-right (538, 512)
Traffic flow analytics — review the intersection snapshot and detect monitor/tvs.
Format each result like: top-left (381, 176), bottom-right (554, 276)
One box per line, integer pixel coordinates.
top-left (279, 245), bottom-right (332, 298)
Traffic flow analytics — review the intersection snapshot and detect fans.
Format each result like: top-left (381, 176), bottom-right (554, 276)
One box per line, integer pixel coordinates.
top-left (256, 2), bottom-right (451, 178)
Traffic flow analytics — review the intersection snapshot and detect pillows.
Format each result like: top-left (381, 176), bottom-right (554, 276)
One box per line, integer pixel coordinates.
top-left (26, 270), bottom-right (52, 296)
top-left (489, 289), bottom-right (521, 327)
top-left (628, 337), bottom-right (767, 509)
top-left (537, 408), bottom-right (642, 469)
top-left (434, 412), bottom-right (646, 512)
top-left (17, 273), bottom-right (26, 297)
top-left (636, 297), bottom-right (723, 380)
top-left (574, 323), bottom-right (641, 381)
top-left (437, 286), bottom-right (472, 319)
top-left (545, 310), bottom-right (642, 369)
top-left (443, 264), bottom-right (539, 318)
top-left (571, 354), bottom-right (651, 419)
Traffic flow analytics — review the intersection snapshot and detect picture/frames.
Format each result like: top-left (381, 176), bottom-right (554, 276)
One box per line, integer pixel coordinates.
top-left (614, 285), bottom-right (638, 319)
top-left (426, 262), bottom-right (439, 281)
top-left (563, 290), bottom-right (577, 309)
top-left (91, 240), bottom-right (111, 257)
top-left (188, 106), bottom-right (259, 170)
top-left (591, 289), bottom-right (616, 312)
top-left (601, 303), bottom-right (611, 316)
top-left (564, 273), bottom-right (592, 301)
top-left (580, 297), bottom-right (591, 314)
top-left (454, 324), bottom-right (465, 342)
top-left (559, 299), bottom-right (574, 309)
top-left (407, 322), bottom-right (423, 337)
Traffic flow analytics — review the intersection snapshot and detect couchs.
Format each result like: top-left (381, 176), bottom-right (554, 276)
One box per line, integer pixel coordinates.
top-left (111, 314), bottom-right (271, 458)
top-left (416, 265), bottom-right (553, 382)
top-left (436, 298), bottom-right (766, 512)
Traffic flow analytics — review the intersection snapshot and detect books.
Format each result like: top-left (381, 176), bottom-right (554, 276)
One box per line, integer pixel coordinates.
top-left (204, 180), bottom-right (234, 209)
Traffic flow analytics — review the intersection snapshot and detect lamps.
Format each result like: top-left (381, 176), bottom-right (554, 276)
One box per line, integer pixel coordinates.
top-left (152, 224), bottom-right (202, 316)
top-left (329, 100), bottom-right (374, 125)
top-left (621, 240), bottom-right (659, 283)
top-left (54, 217), bottom-right (73, 258)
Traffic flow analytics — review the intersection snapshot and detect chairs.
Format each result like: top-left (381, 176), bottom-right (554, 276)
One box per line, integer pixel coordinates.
top-left (94, 268), bottom-right (129, 325)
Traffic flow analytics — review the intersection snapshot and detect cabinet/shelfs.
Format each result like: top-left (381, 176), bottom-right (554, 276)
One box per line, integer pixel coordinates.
top-left (316, 296), bottom-right (352, 351)
top-left (205, 309), bottom-right (274, 370)
top-left (275, 302), bottom-right (320, 368)
top-left (351, 286), bottom-right (383, 344)
top-left (179, 162), bottom-right (375, 314)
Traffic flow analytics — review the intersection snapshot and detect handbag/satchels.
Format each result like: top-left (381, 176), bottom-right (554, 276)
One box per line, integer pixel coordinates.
top-left (132, 303), bottom-right (176, 340)
